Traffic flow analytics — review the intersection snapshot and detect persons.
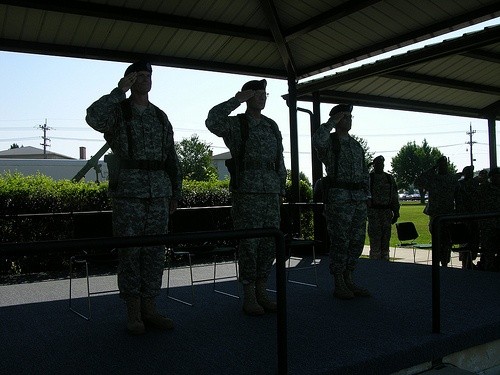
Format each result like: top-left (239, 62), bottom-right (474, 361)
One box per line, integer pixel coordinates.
top-left (86, 63), bottom-right (183, 334)
top-left (366, 156), bottom-right (400, 265)
top-left (206, 79), bottom-right (287, 315)
top-left (312, 103), bottom-right (371, 299)
top-left (415, 156), bottom-right (500, 269)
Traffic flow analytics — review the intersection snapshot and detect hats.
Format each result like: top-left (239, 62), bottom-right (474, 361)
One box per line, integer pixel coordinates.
top-left (463, 166), bottom-right (473, 172)
top-left (479, 169), bottom-right (487, 176)
top-left (373, 156), bottom-right (384, 163)
top-left (329, 104), bottom-right (353, 116)
top-left (437, 156), bottom-right (447, 165)
top-left (242, 79), bottom-right (267, 91)
top-left (125, 61), bottom-right (152, 75)
top-left (489, 167), bottom-right (500, 175)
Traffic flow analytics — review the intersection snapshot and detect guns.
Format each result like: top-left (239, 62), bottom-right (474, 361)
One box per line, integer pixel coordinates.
top-left (225, 159), bottom-right (241, 190)
top-left (104, 155), bottom-right (123, 193)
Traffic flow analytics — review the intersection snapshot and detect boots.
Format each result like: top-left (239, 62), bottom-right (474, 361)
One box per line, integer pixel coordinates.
top-left (127, 297), bottom-right (145, 335)
top-left (143, 298), bottom-right (172, 327)
top-left (344, 267), bottom-right (359, 294)
top-left (243, 283), bottom-right (264, 316)
top-left (462, 260), bottom-right (476, 270)
top-left (255, 281), bottom-right (274, 310)
top-left (329, 268), bottom-right (354, 300)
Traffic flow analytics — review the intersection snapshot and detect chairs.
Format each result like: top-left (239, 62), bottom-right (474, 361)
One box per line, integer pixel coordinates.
top-left (396, 222), bottom-right (433, 249)
top-left (164, 208), bottom-right (240, 306)
top-left (69, 212), bottom-right (120, 321)
top-left (254, 204), bottom-right (321, 293)
